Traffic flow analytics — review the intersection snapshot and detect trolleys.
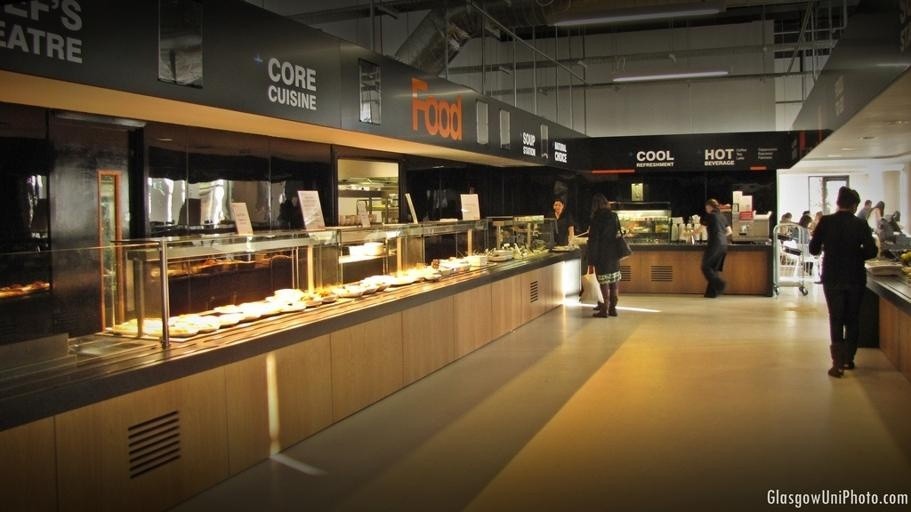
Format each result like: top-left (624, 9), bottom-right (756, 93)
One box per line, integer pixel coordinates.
top-left (773, 223), bottom-right (810, 298)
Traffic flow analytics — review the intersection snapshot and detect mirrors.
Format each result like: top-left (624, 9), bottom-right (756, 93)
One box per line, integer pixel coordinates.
top-left (96, 170), bottom-right (126, 332)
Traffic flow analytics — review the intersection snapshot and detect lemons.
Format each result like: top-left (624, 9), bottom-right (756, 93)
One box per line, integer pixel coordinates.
top-left (901, 251), bottom-right (911, 262)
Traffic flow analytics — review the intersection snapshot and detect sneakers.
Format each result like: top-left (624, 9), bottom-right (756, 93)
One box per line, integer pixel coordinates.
top-left (592, 300), bottom-right (603, 312)
top-left (592, 310), bottom-right (607, 318)
top-left (609, 308), bottom-right (619, 317)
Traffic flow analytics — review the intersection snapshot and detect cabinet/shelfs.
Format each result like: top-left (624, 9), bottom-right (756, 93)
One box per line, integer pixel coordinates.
top-left (878, 294), bottom-right (911, 382)
top-left (580, 243), bottom-right (772, 295)
top-left (1, 247), bottom-right (582, 511)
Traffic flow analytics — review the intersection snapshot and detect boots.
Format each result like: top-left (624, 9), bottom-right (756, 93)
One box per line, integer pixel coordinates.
top-left (827, 342), bottom-right (846, 378)
top-left (841, 340), bottom-right (858, 371)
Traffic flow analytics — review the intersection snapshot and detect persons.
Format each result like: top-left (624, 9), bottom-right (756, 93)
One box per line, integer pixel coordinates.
top-left (588, 191), bottom-right (622, 318)
top-left (810, 186), bottom-right (880, 379)
top-left (782, 196), bottom-right (907, 262)
top-left (700, 197), bottom-right (734, 299)
top-left (545, 198), bottom-right (575, 248)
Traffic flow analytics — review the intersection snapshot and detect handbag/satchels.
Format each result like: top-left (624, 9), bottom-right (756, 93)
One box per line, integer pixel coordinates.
top-left (614, 232), bottom-right (633, 260)
top-left (578, 271), bottom-right (604, 305)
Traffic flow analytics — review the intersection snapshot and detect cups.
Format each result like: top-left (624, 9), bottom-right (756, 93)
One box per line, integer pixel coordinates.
top-left (671, 222), bottom-right (708, 245)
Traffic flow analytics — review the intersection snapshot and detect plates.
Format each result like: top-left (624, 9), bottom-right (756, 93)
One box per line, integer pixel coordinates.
top-left (106, 254), bottom-right (471, 339)
top-left (550, 245), bottom-right (573, 252)
top-left (488, 250), bottom-right (514, 263)
top-left (465, 255), bottom-right (489, 267)
top-left (364, 242), bottom-right (385, 256)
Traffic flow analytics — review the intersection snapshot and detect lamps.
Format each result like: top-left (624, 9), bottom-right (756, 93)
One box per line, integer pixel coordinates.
top-left (612, 71), bottom-right (729, 84)
top-left (550, 8), bottom-right (721, 27)
